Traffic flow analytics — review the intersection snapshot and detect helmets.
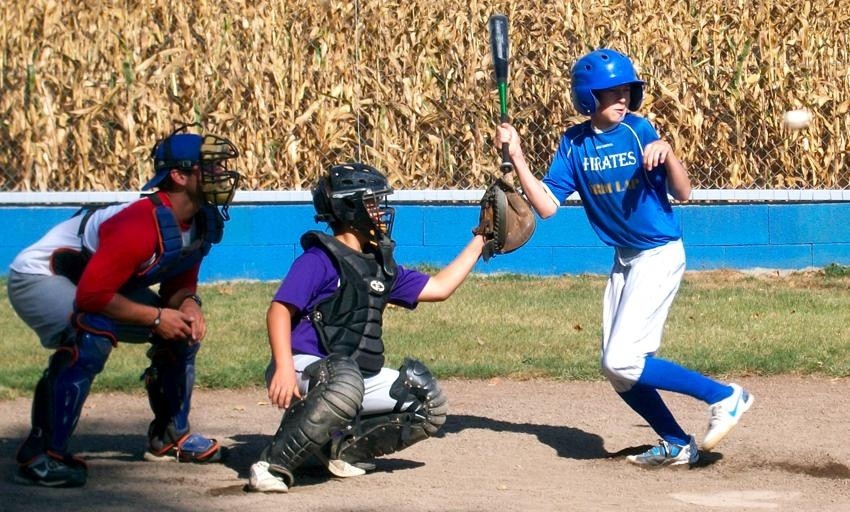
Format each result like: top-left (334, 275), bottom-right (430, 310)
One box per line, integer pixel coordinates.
top-left (571, 49), bottom-right (645, 116)
top-left (314, 162), bottom-right (395, 243)
top-left (142, 123), bottom-right (240, 221)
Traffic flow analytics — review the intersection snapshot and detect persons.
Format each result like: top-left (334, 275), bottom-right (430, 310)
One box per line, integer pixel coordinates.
top-left (7, 133), bottom-right (245, 487)
top-left (250, 163), bottom-right (535, 492)
top-left (495, 48), bottom-right (753, 470)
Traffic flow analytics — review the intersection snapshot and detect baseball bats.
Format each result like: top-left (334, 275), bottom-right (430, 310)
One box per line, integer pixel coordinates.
top-left (490, 13), bottom-right (513, 172)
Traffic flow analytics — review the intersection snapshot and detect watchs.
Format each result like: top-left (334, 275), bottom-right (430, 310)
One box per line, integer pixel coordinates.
top-left (180, 295), bottom-right (201, 308)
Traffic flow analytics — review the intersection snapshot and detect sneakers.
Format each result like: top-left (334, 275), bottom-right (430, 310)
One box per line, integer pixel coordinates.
top-left (14, 453), bottom-right (86, 486)
top-left (249, 461), bottom-right (289, 493)
top-left (316, 450), bottom-right (366, 477)
top-left (626, 434), bottom-right (699, 465)
top-left (144, 437), bottom-right (228, 461)
top-left (701, 383), bottom-right (755, 451)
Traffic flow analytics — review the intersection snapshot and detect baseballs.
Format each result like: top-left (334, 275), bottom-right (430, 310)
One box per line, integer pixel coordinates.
top-left (785, 110), bottom-right (811, 131)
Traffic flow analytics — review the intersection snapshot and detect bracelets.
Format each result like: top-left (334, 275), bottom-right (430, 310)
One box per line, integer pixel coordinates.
top-left (149, 308), bottom-right (162, 331)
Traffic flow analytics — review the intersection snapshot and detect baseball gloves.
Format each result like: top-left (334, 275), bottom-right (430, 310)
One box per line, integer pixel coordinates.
top-left (473, 178), bottom-right (536, 261)
